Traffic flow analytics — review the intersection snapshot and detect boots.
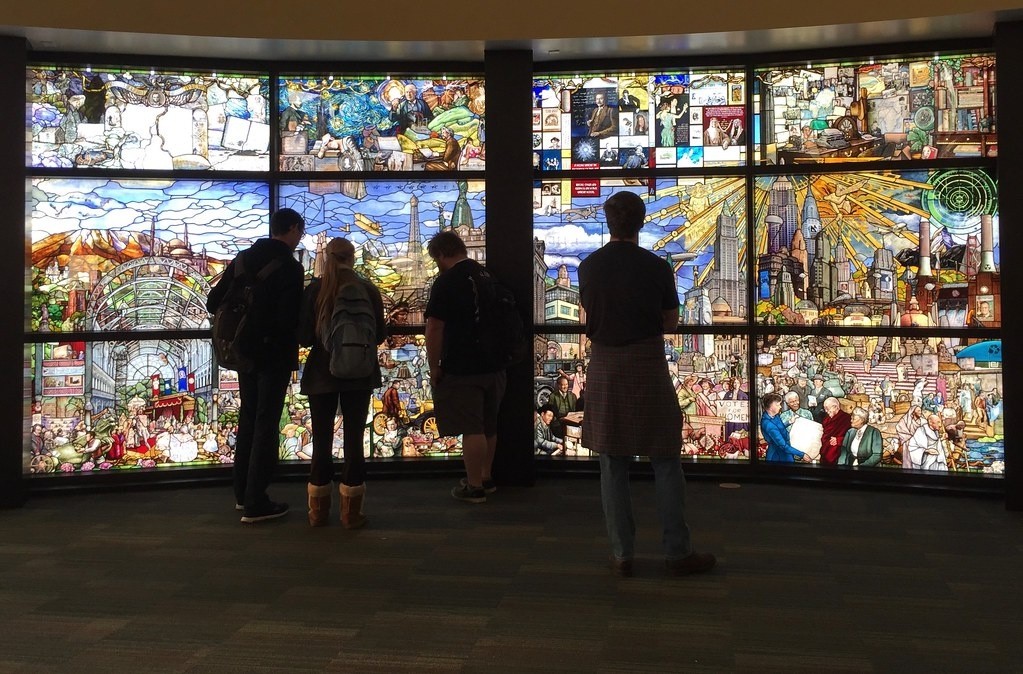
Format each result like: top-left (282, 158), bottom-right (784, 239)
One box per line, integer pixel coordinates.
top-left (307, 483), bottom-right (332, 528)
top-left (339, 484), bottom-right (366, 528)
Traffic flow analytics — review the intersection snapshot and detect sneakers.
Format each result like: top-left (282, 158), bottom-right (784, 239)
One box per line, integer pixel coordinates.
top-left (451, 478), bottom-right (497, 504)
top-left (670, 552), bottom-right (716, 578)
top-left (235, 496), bottom-right (289, 522)
top-left (609, 552), bottom-right (636, 578)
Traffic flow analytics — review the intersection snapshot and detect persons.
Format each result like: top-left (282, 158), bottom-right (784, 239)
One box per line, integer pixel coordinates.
top-left (296, 237), bottom-right (388, 528)
top-left (578, 191), bottom-right (718, 576)
top-left (424, 232), bottom-right (508, 503)
top-left (206, 208), bottom-right (304, 526)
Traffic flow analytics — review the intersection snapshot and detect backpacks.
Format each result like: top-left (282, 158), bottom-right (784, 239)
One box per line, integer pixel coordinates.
top-left (441, 273), bottom-right (528, 370)
top-left (329, 282), bottom-right (374, 377)
top-left (210, 252), bottom-right (285, 371)
